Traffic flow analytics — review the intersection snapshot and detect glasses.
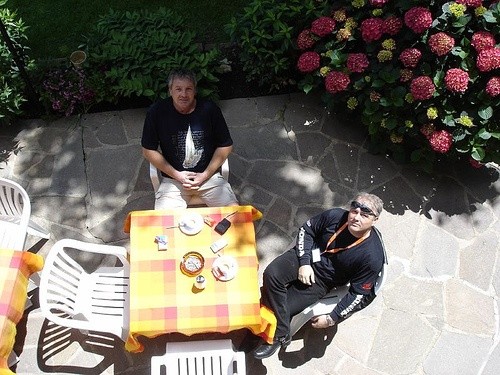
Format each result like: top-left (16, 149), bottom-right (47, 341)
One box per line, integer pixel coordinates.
top-left (350, 200), bottom-right (377, 217)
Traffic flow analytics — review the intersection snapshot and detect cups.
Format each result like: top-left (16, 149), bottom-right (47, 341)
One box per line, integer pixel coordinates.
top-left (217, 262), bottom-right (230, 275)
top-left (184, 219), bottom-right (196, 228)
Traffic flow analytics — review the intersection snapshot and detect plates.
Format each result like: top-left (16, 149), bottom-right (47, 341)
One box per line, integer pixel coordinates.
top-left (212, 255), bottom-right (238, 281)
top-left (179, 212), bottom-right (204, 235)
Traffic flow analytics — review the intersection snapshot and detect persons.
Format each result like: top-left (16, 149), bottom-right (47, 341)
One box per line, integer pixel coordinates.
top-left (253, 192), bottom-right (385, 359)
top-left (141, 69), bottom-right (239, 211)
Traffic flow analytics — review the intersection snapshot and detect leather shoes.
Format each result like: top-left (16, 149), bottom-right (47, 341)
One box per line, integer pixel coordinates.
top-left (239, 331), bottom-right (257, 353)
top-left (254, 334), bottom-right (292, 359)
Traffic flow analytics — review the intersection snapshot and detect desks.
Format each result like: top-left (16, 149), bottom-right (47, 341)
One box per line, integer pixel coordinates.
top-left (0, 221), bottom-right (44, 374)
top-left (124, 205), bottom-right (278, 353)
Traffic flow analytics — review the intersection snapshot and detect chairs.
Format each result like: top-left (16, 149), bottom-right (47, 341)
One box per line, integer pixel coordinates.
top-left (150, 339), bottom-right (246, 375)
top-left (0, 176), bottom-right (50, 239)
top-left (39, 239), bottom-right (130, 344)
top-left (150, 158), bottom-right (229, 205)
top-left (280, 235), bottom-right (385, 335)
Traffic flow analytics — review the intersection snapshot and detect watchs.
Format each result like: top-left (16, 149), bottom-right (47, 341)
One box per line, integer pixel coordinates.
top-left (325, 314), bottom-right (335, 327)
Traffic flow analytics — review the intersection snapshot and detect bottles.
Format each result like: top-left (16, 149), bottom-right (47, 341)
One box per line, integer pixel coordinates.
top-left (195, 275), bottom-right (205, 290)
